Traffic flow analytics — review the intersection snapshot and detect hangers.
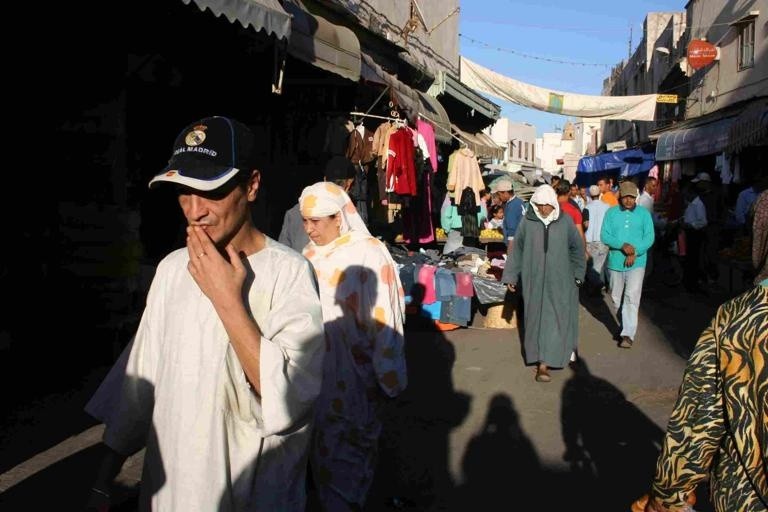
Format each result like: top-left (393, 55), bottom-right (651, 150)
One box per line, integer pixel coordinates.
top-left (342, 112), bottom-right (424, 136)
top-left (460, 143), bottom-right (475, 155)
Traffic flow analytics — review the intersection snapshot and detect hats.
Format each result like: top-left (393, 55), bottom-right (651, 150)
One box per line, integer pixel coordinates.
top-left (589, 185), bottom-right (599, 196)
top-left (618, 182), bottom-right (637, 198)
top-left (489, 180), bottom-right (512, 193)
top-left (146, 116), bottom-right (257, 192)
top-left (691, 172), bottom-right (711, 183)
top-left (325, 155), bottom-right (355, 179)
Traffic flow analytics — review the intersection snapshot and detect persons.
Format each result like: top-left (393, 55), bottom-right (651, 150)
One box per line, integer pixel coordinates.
top-left (84, 116), bottom-right (325, 511)
top-left (475, 168), bottom-right (768, 384)
top-left (277, 152), bottom-right (359, 255)
top-left (300, 181), bottom-right (412, 511)
top-left (629, 190), bottom-right (768, 511)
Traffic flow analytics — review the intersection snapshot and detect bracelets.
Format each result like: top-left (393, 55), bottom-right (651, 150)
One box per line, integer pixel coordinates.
top-left (88, 478), bottom-right (114, 502)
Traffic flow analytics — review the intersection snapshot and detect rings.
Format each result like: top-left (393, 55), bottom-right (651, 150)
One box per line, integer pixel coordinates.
top-left (198, 252), bottom-right (207, 259)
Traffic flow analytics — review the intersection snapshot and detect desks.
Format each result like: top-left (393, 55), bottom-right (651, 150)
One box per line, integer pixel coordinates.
top-left (718, 247), bottom-right (760, 296)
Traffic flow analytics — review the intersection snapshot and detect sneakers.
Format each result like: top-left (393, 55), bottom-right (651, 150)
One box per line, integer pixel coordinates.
top-left (619, 337), bottom-right (631, 347)
top-left (535, 371), bottom-right (550, 382)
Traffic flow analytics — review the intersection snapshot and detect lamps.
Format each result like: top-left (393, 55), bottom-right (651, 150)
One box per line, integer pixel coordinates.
top-left (654, 42), bottom-right (674, 59)
